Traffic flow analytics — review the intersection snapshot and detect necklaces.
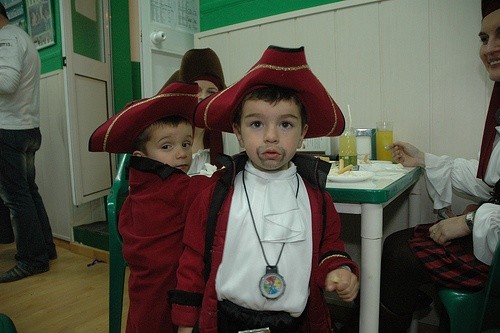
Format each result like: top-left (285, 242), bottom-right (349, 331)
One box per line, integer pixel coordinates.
top-left (242, 169), bottom-right (300, 301)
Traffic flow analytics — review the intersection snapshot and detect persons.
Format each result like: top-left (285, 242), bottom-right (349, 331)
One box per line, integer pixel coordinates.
top-left (0, 3), bottom-right (57, 282)
top-left (169, 44), bottom-right (362, 333)
top-left (350, 0), bottom-right (500, 333)
top-left (161, 47), bottom-right (228, 176)
top-left (87, 80), bottom-right (210, 332)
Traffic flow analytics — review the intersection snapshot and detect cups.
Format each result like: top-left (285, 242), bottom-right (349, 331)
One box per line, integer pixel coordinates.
top-left (339, 127), bottom-right (357, 168)
top-left (376, 119), bottom-right (394, 161)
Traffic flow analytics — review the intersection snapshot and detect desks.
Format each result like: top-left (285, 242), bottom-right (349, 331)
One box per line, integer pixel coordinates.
top-left (325, 166), bottom-right (422, 333)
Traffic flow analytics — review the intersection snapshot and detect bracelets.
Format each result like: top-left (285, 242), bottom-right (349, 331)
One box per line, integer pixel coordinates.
top-left (465, 212), bottom-right (474, 232)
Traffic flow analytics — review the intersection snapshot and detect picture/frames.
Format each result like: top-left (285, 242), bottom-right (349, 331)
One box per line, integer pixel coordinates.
top-left (24, 0), bottom-right (56, 51)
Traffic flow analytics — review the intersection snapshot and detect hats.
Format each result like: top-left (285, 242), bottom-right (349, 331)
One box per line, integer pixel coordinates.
top-left (87, 82), bottom-right (200, 155)
top-left (158, 47), bottom-right (227, 93)
top-left (192, 44), bottom-right (346, 139)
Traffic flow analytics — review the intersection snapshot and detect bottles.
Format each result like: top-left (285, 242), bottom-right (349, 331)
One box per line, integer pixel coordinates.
top-left (356, 129), bottom-right (372, 160)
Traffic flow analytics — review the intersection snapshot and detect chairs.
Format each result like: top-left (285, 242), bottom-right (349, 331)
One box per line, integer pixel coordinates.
top-left (437, 240), bottom-right (500, 333)
top-left (107, 151), bottom-right (129, 333)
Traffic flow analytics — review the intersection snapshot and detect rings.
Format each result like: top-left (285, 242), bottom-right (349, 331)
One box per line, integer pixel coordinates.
top-left (432, 230), bottom-right (436, 234)
